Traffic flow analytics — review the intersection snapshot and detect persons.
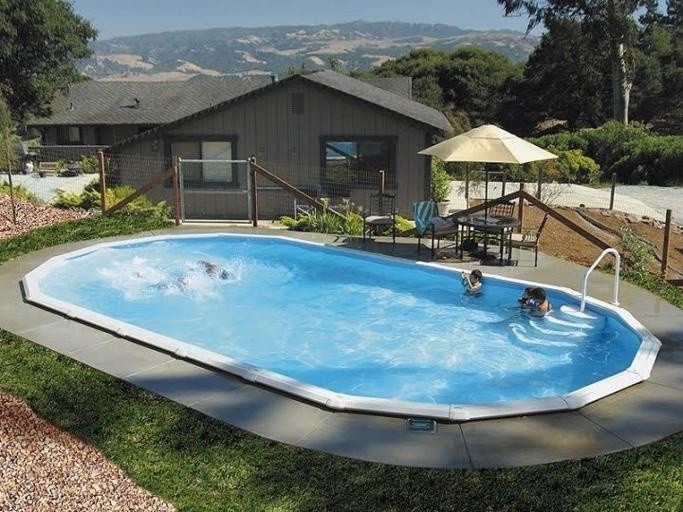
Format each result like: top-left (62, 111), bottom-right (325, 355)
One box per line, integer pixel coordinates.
top-left (531, 287), bottom-right (552, 316)
top-left (460, 269), bottom-right (482, 297)
top-left (520, 287), bottom-right (536, 309)
top-left (134, 261), bottom-right (233, 292)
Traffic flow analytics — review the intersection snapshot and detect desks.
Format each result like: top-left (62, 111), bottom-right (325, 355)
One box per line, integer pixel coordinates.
top-left (453, 212), bottom-right (520, 267)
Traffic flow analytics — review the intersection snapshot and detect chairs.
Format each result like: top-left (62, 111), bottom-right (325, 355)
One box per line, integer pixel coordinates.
top-left (411, 198), bottom-right (460, 261)
top-left (482, 196), bottom-right (516, 266)
top-left (360, 189), bottom-right (399, 250)
top-left (505, 209), bottom-right (550, 269)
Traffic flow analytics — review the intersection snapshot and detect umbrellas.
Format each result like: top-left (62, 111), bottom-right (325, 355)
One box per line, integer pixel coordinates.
top-left (416, 124), bottom-right (560, 261)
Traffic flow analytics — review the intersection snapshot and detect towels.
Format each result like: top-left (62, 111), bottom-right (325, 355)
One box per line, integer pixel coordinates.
top-left (410, 199), bottom-right (438, 237)
